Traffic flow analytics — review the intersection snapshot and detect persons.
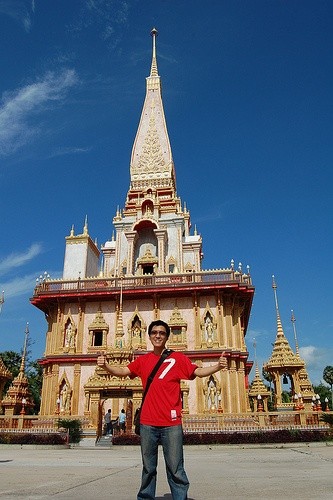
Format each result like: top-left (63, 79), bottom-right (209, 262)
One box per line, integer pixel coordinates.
top-left (104, 409), bottom-right (112, 436)
top-left (96, 320), bottom-right (227, 500)
top-left (118, 409), bottom-right (126, 432)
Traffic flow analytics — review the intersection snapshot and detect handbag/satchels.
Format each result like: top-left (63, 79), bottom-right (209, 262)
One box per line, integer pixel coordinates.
top-left (134, 408), bottom-right (141, 435)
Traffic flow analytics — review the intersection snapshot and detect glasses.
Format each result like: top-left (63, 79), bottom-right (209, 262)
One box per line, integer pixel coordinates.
top-left (150, 330), bottom-right (166, 336)
